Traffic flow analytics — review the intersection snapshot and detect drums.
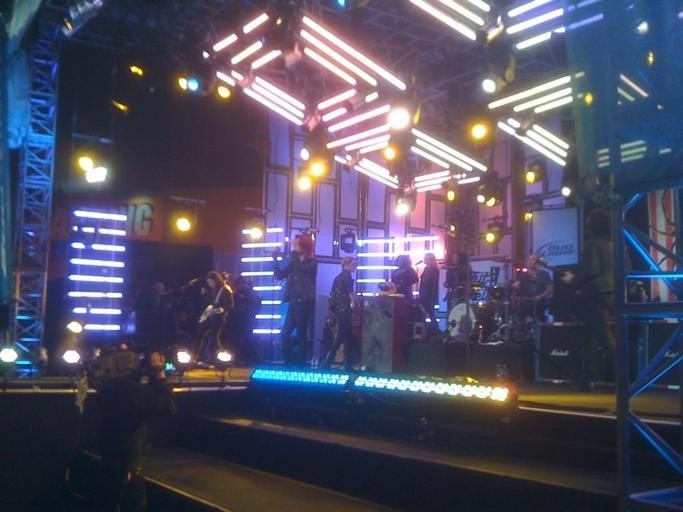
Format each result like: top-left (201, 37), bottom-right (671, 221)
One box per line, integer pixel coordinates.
top-left (493, 285), bottom-right (521, 306)
top-left (447, 302), bottom-right (487, 337)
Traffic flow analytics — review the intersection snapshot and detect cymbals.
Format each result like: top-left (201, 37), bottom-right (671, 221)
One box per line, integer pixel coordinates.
top-left (459, 284), bottom-right (483, 290)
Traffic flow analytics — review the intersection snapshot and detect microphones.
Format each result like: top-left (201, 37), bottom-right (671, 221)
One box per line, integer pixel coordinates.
top-left (415, 260), bottom-right (423, 265)
top-left (539, 257), bottom-right (550, 268)
top-left (188, 274), bottom-right (201, 286)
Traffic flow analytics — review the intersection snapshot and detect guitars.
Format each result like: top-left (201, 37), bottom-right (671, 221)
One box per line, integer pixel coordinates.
top-left (198, 305), bottom-right (223, 323)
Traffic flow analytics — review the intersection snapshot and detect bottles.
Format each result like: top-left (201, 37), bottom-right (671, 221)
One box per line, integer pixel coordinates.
top-left (384, 278), bottom-right (390, 291)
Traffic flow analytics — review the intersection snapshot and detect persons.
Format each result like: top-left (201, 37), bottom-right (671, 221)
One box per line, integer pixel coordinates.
top-left (391, 251), bottom-right (467, 321)
top-left (271, 235), bottom-right (361, 372)
top-left (78, 348), bottom-right (178, 511)
top-left (512, 253), bottom-right (613, 351)
top-left (135, 271), bottom-right (262, 367)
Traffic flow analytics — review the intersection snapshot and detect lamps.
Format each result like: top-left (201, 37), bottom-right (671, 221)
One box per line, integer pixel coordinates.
top-left (442, 156), bottom-right (547, 244)
top-left (474, 12), bottom-right (517, 94)
